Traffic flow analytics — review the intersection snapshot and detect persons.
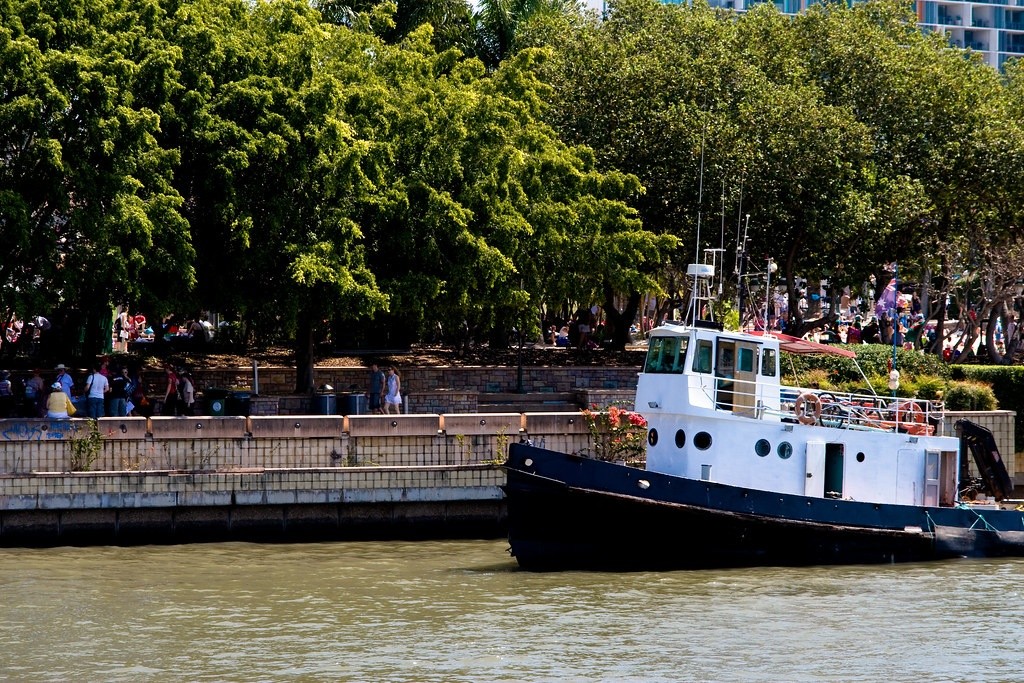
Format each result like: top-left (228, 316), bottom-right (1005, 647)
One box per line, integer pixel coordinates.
top-left (804, 291), bottom-right (925, 345)
top-left (648, 316), bottom-right (653, 330)
top-left (548, 301), bottom-right (597, 345)
top-left (187, 315), bottom-right (214, 340)
top-left (46, 363), bottom-right (73, 418)
top-left (968, 304), bottom-right (978, 333)
top-left (115, 307), bottom-right (130, 353)
top-left (164, 366), bottom-right (194, 416)
top-left (25, 368), bottom-right (43, 404)
top-left (994, 313), bottom-right (1004, 348)
top-left (0, 370), bottom-right (13, 403)
top-left (30, 315), bottom-right (51, 344)
top-left (85, 365), bottom-right (133, 417)
top-left (370, 363), bottom-right (402, 415)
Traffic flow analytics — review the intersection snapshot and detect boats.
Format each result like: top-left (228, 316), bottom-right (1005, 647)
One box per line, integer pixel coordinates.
top-left (493, 90), bottom-right (1024, 573)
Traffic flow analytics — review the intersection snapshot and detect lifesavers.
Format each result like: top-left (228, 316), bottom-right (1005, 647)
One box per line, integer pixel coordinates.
top-left (794, 394), bottom-right (821, 425)
top-left (895, 403), bottom-right (923, 431)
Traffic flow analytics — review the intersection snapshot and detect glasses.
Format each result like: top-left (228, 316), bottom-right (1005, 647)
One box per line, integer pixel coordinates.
top-left (387, 369), bottom-right (393, 371)
top-left (57, 369), bottom-right (63, 372)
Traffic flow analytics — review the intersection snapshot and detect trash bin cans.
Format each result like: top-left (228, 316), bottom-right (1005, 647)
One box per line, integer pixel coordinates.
top-left (202, 388), bottom-right (228, 416)
top-left (315, 384), bottom-right (337, 415)
top-left (345, 384), bottom-right (367, 415)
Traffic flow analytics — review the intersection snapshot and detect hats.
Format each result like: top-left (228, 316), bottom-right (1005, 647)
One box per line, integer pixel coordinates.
top-left (54, 364), bottom-right (70, 371)
top-left (51, 382), bottom-right (62, 389)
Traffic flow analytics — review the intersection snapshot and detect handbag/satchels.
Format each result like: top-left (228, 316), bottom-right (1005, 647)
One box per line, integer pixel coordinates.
top-left (85, 373), bottom-right (94, 396)
top-left (66, 394), bottom-right (76, 415)
top-left (175, 381), bottom-right (182, 403)
